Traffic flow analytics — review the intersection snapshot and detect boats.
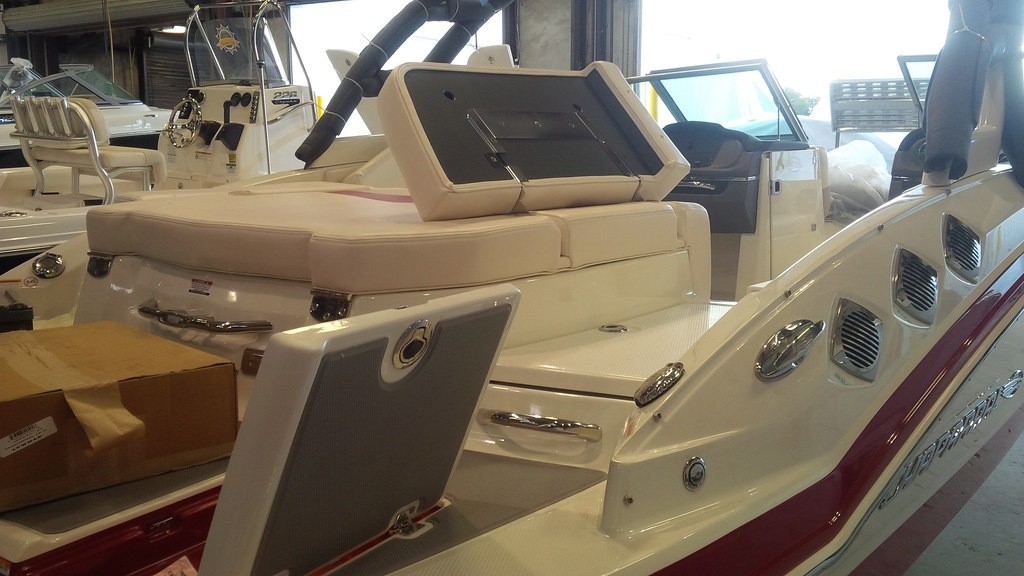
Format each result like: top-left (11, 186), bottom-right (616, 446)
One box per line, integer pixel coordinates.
top-left (1, 57), bottom-right (180, 168)
top-left (0, 0), bottom-right (318, 275)
top-left (1, 0), bottom-right (1024, 574)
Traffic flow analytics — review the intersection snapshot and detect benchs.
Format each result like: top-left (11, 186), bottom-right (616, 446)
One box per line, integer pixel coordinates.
top-left (830, 77), bottom-right (927, 148)
top-left (8, 95), bottom-right (167, 203)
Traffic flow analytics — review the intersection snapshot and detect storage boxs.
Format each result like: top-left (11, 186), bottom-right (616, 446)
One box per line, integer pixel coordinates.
top-left (0, 320), bottom-right (240, 517)
